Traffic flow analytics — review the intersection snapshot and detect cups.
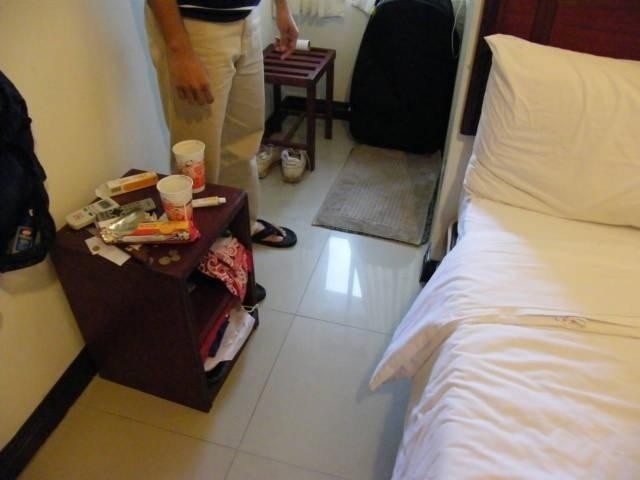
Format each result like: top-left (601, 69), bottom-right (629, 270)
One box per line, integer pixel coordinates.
top-left (172, 139), bottom-right (206, 193)
top-left (156, 174), bottom-right (193, 224)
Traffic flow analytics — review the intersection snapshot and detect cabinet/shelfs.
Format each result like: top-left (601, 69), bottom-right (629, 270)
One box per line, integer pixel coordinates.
top-left (47, 167), bottom-right (260, 414)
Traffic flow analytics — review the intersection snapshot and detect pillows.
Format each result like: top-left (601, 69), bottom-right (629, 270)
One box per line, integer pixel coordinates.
top-left (463, 29), bottom-right (639, 225)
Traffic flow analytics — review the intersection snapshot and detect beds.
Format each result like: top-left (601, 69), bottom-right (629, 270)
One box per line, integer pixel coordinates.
top-left (397, 189), bottom-right (639, 480)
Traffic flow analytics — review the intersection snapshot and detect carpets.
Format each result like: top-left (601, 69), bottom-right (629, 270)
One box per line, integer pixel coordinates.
top-left (310, 142), bottom-right (443, 248)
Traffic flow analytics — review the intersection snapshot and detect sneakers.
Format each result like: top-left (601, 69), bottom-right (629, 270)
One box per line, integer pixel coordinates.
top-left (257, 144), bottom-right (282, 179)
top-left (280, 147), bottom-right (308, 183)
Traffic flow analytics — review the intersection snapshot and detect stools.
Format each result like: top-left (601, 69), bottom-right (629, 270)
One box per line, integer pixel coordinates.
top-left (261, 42), bottom-right (337, 171)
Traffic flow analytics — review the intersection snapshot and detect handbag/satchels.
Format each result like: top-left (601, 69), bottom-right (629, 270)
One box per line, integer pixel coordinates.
top-left (0, 70), bottom-right (55, 272)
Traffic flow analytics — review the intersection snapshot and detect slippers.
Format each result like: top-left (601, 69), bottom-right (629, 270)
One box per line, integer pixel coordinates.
top-left (251, 219), bottom-right (297, 248)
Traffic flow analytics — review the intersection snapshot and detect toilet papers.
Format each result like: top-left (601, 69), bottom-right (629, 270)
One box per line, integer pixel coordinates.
top-left (296, 39), bottom-right (311, 51)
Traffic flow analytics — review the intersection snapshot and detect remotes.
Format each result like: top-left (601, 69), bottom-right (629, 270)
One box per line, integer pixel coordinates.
top-left (95, 198), bottom-right (156, 223)
top-left (64, 197), bottom-right (120, 230)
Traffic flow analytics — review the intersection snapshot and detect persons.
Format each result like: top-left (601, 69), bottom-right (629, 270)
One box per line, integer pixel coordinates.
top-left (143, 0), bottom-right (302, 306)
